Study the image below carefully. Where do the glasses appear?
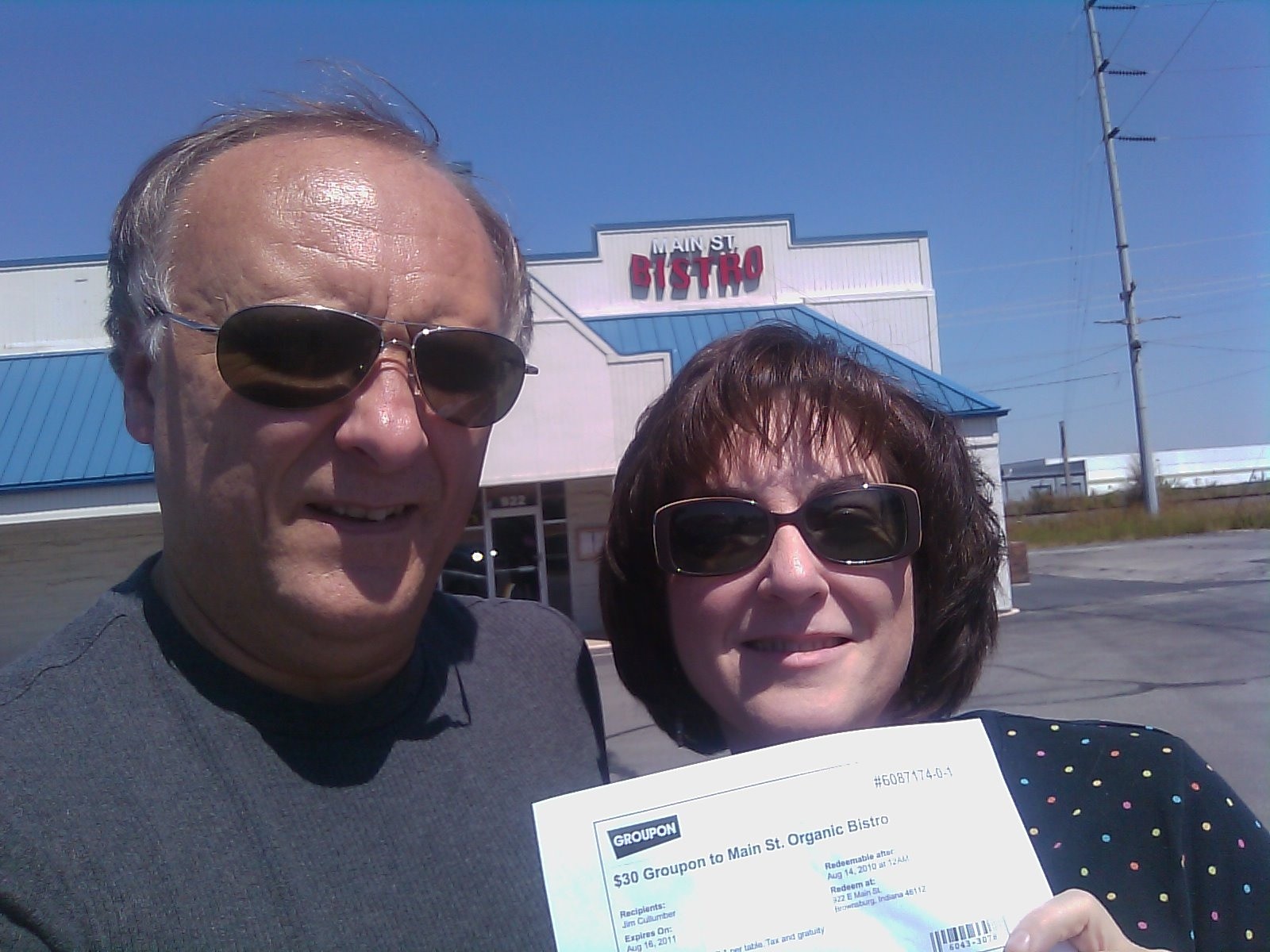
[144,292,537,428]
[646,484,923,578]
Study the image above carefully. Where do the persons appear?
[601,317,1270,952]
[0,68,612,952]
